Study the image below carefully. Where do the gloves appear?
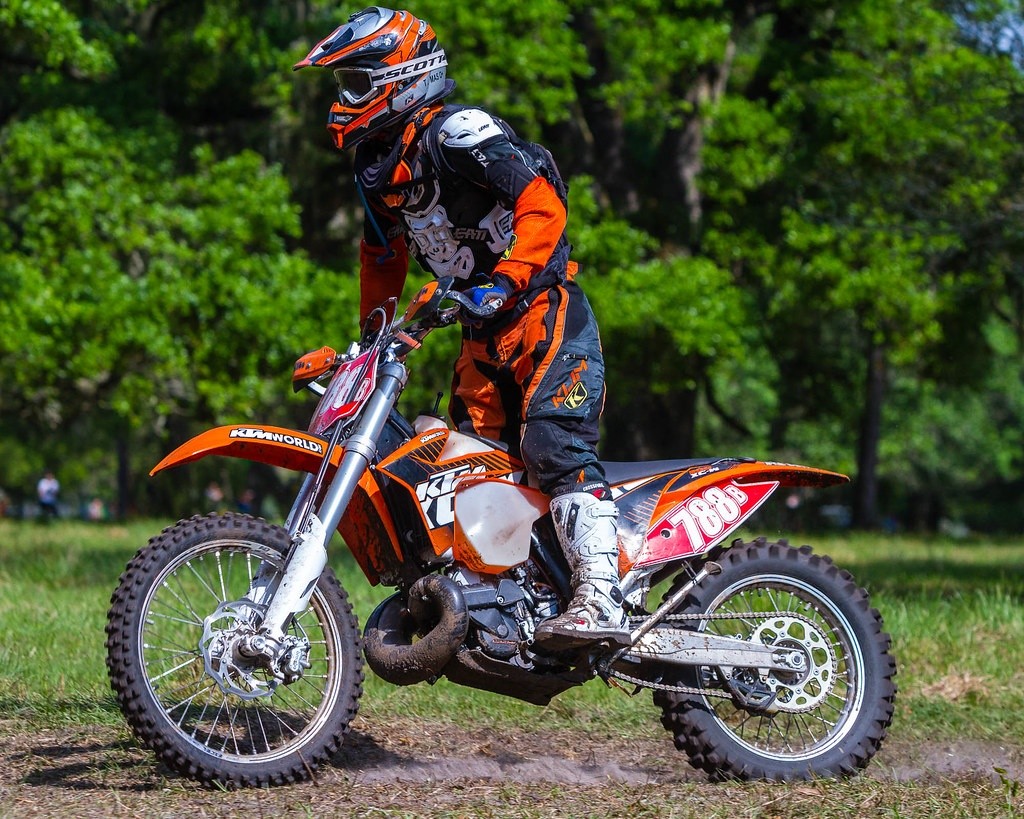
[456,275,516,326]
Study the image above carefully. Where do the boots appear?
[533,492,631,657]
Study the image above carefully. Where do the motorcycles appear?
[105,279,895,791]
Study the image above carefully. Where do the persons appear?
[289,4,633,657]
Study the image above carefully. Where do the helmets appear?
[294,6,447,154]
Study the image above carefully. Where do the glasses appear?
[333,68,381,107]
